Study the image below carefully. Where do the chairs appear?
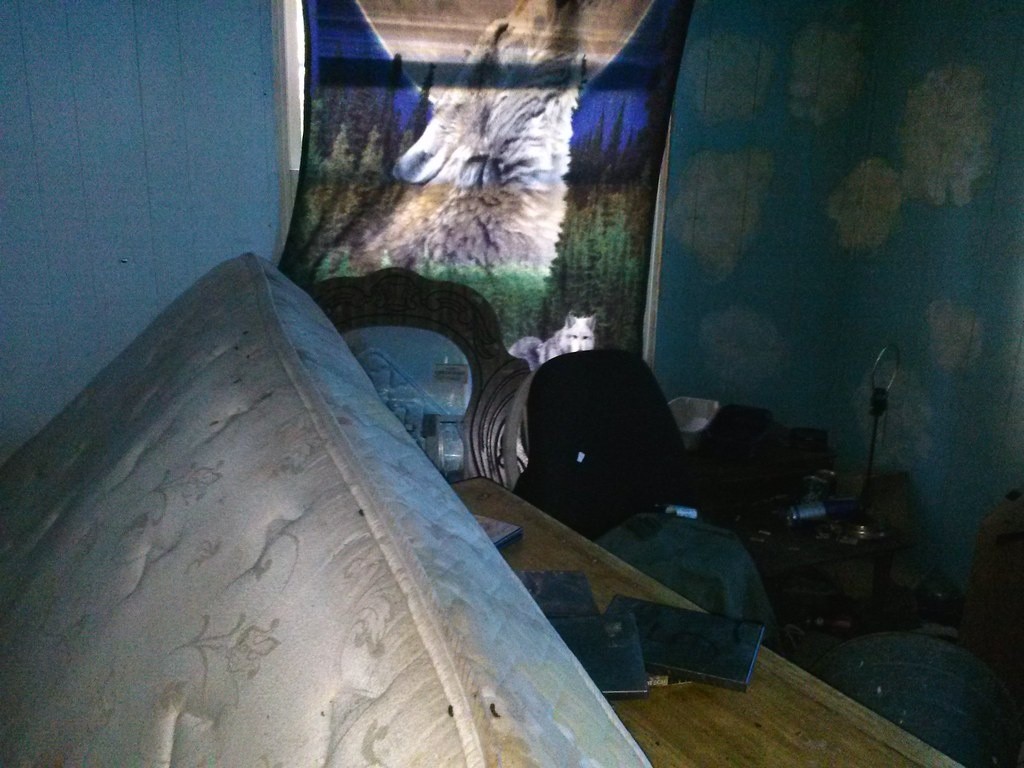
[527,348,691,546]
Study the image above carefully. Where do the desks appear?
[691,451,915,573]
[447,476,968,767]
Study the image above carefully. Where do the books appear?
[473,513,524,547]
[515,568,764,699]
